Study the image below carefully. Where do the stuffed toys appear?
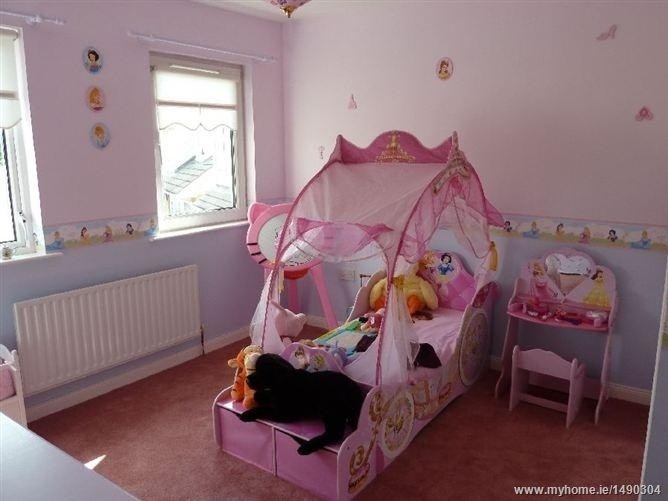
[371,255,440,316]
[227,344,263,409]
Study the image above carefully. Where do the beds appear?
[313,254,502,475]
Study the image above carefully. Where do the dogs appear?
[239,352,369,456]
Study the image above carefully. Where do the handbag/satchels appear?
[413,342,441,368]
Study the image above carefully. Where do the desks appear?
[494,247,618,424]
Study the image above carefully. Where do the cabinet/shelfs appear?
[212,343,380,501]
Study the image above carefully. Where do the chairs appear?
[509,343,587,427]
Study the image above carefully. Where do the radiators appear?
[13,263,204,400]
